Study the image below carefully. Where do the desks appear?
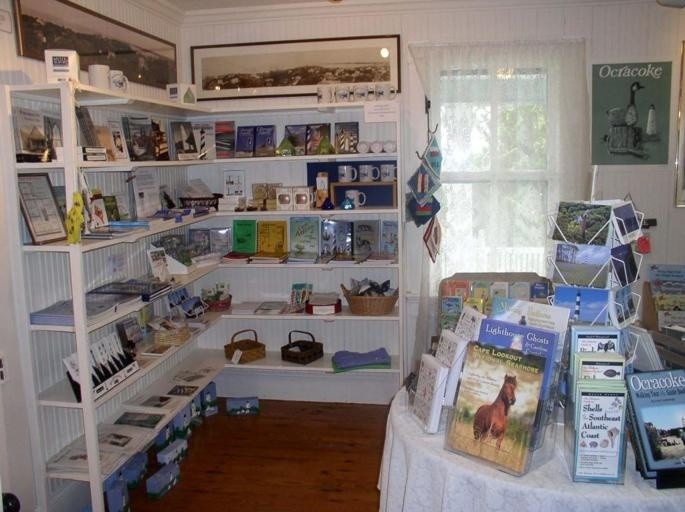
[375,382,685,512]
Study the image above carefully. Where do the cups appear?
[88,64,129,93]
[317,83,397,104]
[338,164,397,209]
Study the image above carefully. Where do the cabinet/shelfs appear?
[2,79,406,512]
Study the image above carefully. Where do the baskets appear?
[280,330,323,365]
[341,283,399,315]
[224,329,265,364]
[154,326,190,346]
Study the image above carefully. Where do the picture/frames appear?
[16,172,67,246]
[12,0,177,89]
[190,33,402,101]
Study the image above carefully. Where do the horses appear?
[471,373,518,456]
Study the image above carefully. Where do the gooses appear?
[604,82,646,127]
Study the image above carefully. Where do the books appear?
[625,326,665,373]
[222,251,397,265]
[11,108,64,163]
[231,301,288,315]
[46,367,214,476]
[215,120,359,158]
[74,108,216,164]
[82,207,210,240]
[411,278,626,486]
[626,368,684,484]
[30,281,173,327]
